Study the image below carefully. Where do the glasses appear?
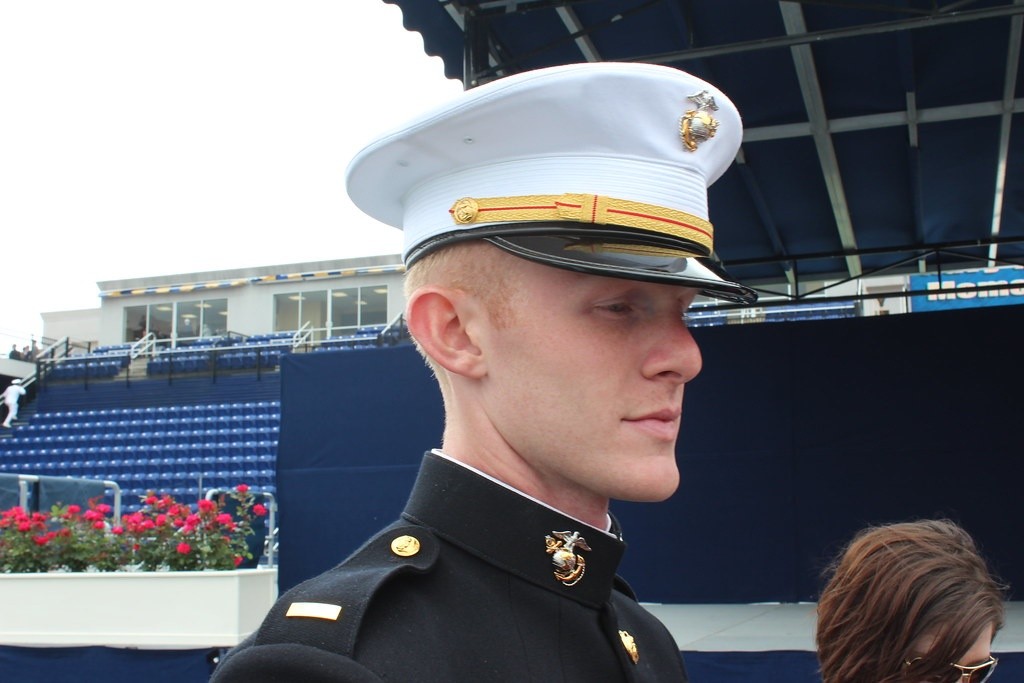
[903,656,999,683]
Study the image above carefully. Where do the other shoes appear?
[13,416,18,419]
[3,423,12,428]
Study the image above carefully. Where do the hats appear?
[11,379,22,384]
[347,59,759,305]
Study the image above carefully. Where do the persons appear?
[815,519,1004,683]
[10,340,38,363]
[207,62,757,683]
[2,379,26,429]
[139,315,211,345]
